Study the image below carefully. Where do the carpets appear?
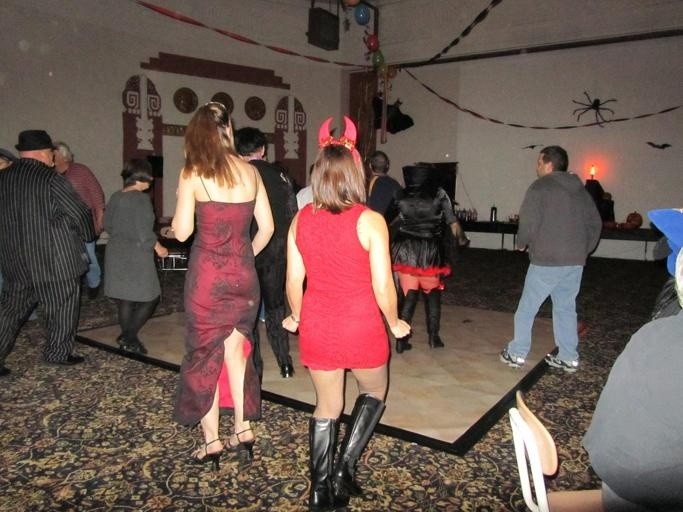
[74,300,590,458]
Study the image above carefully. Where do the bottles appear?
[454,206,477,222]
[490,206,496,222]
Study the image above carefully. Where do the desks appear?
[459,218,663,262]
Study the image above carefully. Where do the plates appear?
[245,96,265,120]
[172,87,197,114]
[210,93,234,115]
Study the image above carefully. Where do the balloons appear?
[347,0,389,75]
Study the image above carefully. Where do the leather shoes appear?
[60,353,82,364]
[279,364,294,378]
[429,337,444,347]
[396,341,412,353]
[116,335,148,354]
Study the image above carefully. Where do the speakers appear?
[306,8,341,51]
[146,155,163,177]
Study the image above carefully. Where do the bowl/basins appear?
[508,215,519,222]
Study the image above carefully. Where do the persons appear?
[579,204,683,512]
[170,101,274,473]
[279,119,412,512]
[388,162,471,354]
[498,144,604,377]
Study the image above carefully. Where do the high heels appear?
[191,439,223,473]
[223,428,256,460]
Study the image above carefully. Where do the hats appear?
[646,203,683,279]
[14,129,57,151]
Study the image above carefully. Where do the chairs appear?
[504,384,562,511]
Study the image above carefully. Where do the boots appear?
[309,417,338,511]
[330,393,387,506]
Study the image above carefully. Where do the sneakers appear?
[499,350,525,368]
[545,353,578,374]
